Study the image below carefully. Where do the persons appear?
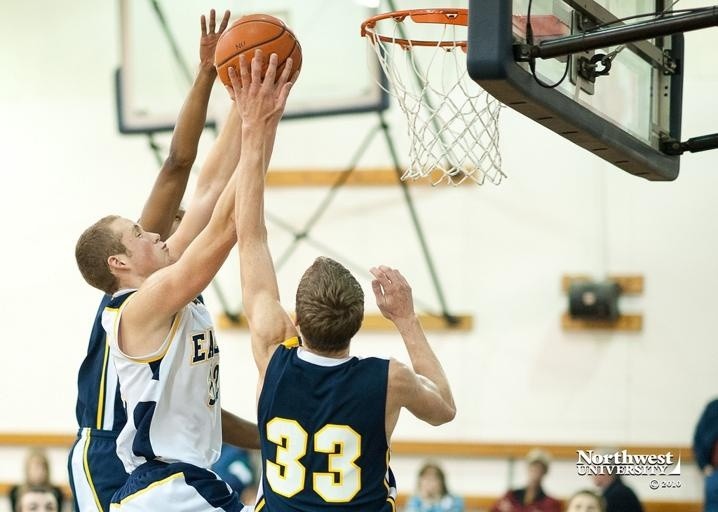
[68,5,263,512]
[72,45,305,512]
[583,448,646,512]
[484,445,565,512]
[562,487,605,512]
[401,458,466,512]
[11,486,62,512]
[6,444,68,512]
[690,398,717,512]
[217,47,461,511]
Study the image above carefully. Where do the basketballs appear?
[215,14,302,88]
